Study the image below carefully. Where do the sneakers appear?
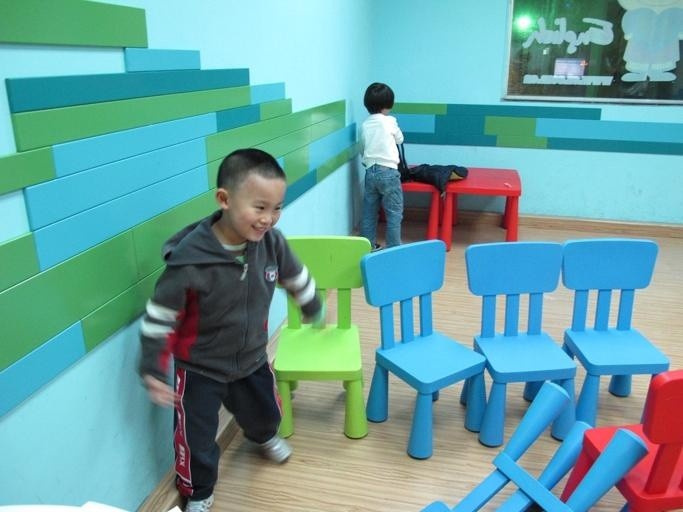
[257,436,292,464]
[371,244,381,252]
[183,493,215,512]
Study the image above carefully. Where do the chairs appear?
[560,241,672,432]
[415,377,648,512]
[360,241,488,463]
[465,240,579,450]
[272,238,372,440]
[555,367,681,512]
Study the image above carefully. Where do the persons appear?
[132,146,326,511]
[355,81,405,253]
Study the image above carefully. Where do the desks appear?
[442,168,520,253]
[377,164,441,241]
